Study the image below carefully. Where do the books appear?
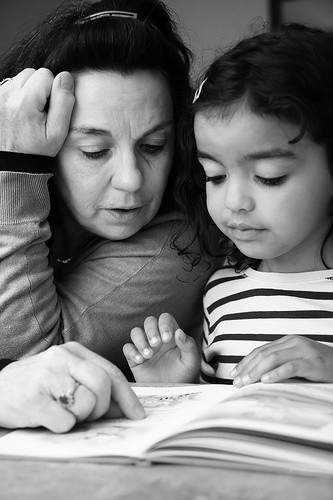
[0,384,333,480]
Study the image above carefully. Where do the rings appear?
[57,380,81,408]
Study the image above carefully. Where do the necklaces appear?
[47,239,78,263]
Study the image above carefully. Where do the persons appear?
[123,22,333,388]
[0,1,233,435]
